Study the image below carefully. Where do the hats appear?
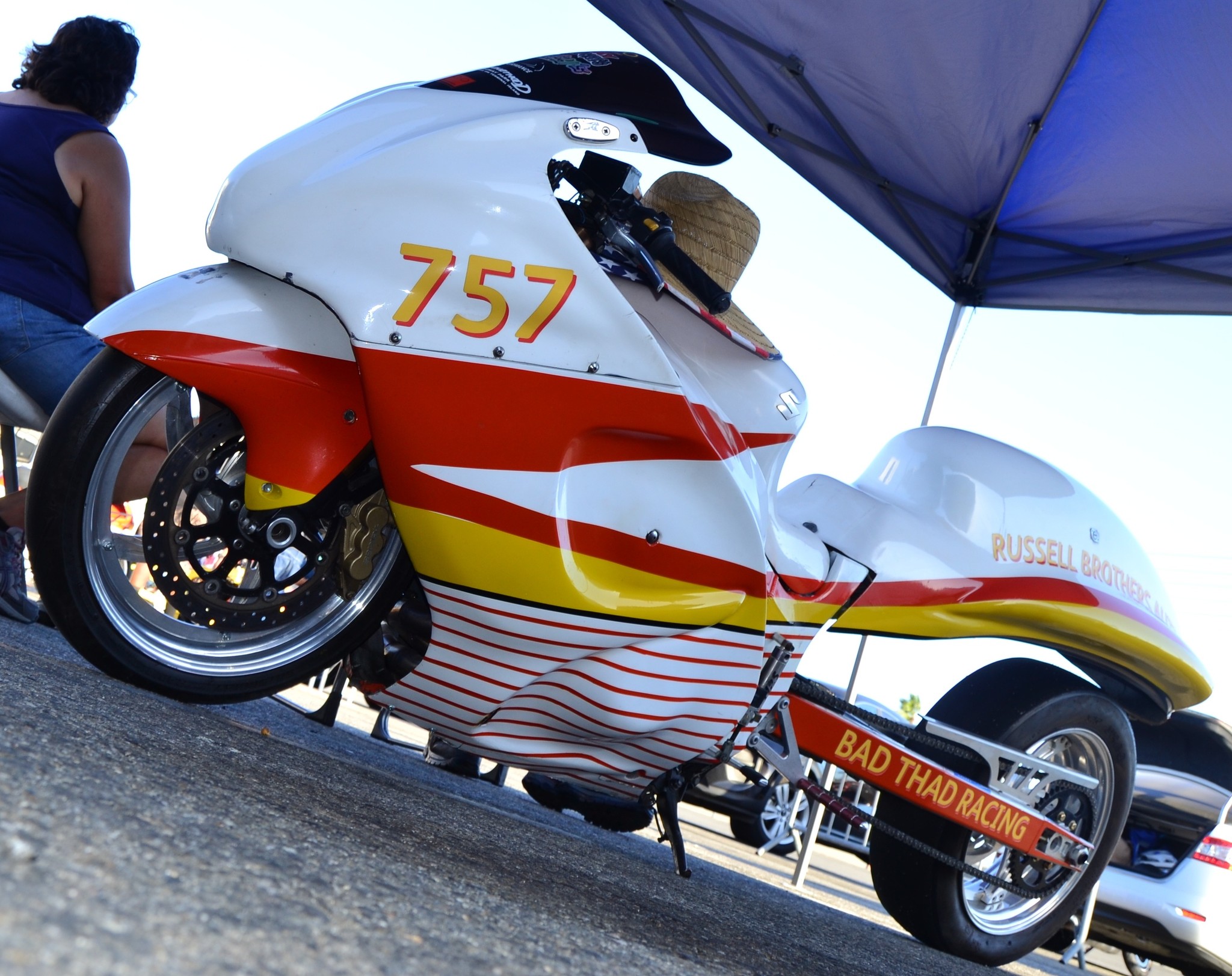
[595,171,784,361]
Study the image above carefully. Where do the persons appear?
[0,17,198,624]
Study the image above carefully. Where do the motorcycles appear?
[19,49,1215,969]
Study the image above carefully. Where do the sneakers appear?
[423,730,481,778]
[0,527,40,624]
[521,771,654,834]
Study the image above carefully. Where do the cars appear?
[1055,708,1232,976]
[695,680,916,856]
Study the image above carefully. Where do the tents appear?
[591,0,1232,890]
